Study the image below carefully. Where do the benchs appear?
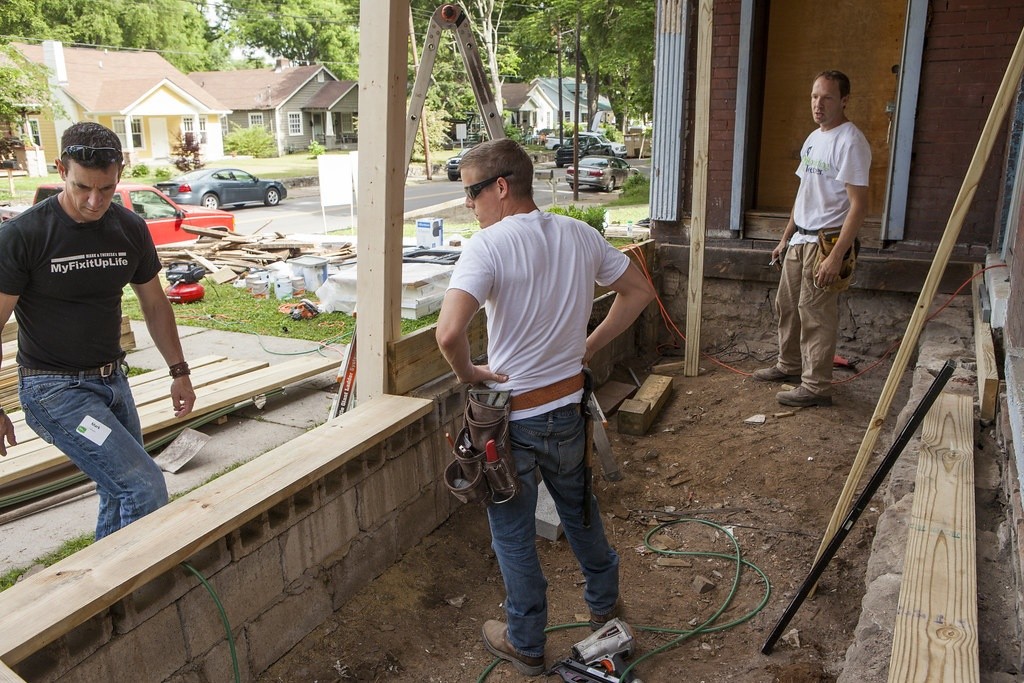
[339,132,358,143]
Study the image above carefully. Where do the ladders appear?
[327,303,357,423]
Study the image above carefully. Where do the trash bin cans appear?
[291,255,330,292]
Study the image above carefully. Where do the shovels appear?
[0,427,212,526]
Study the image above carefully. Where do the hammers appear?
[770,252,783,270]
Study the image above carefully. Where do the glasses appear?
[60,145,124,165]
[463,170,513,200]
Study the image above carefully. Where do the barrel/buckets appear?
[243,268,305,301]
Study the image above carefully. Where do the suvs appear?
[447,142,480,181]
[555,136,613,167]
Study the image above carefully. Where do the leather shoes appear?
[752,365,802,383]
[481,618,546,676]
[589,609,615,632]
[775,385,833,408]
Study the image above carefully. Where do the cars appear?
[155,167,287,209]
[566,154,640,192]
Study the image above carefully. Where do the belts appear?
[17,351,127,378]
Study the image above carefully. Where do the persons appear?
[435,139,652,677]
[0,122,196,542]
[753,70,872,407]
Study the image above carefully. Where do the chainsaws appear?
[289,299,320,321]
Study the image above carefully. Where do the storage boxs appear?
[416,217,443,248]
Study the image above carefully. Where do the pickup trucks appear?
[545,130,627,158]
[32,184,235,245]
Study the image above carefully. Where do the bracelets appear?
[169,362,190,378]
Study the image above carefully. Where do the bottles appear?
[627,220,633,238]
